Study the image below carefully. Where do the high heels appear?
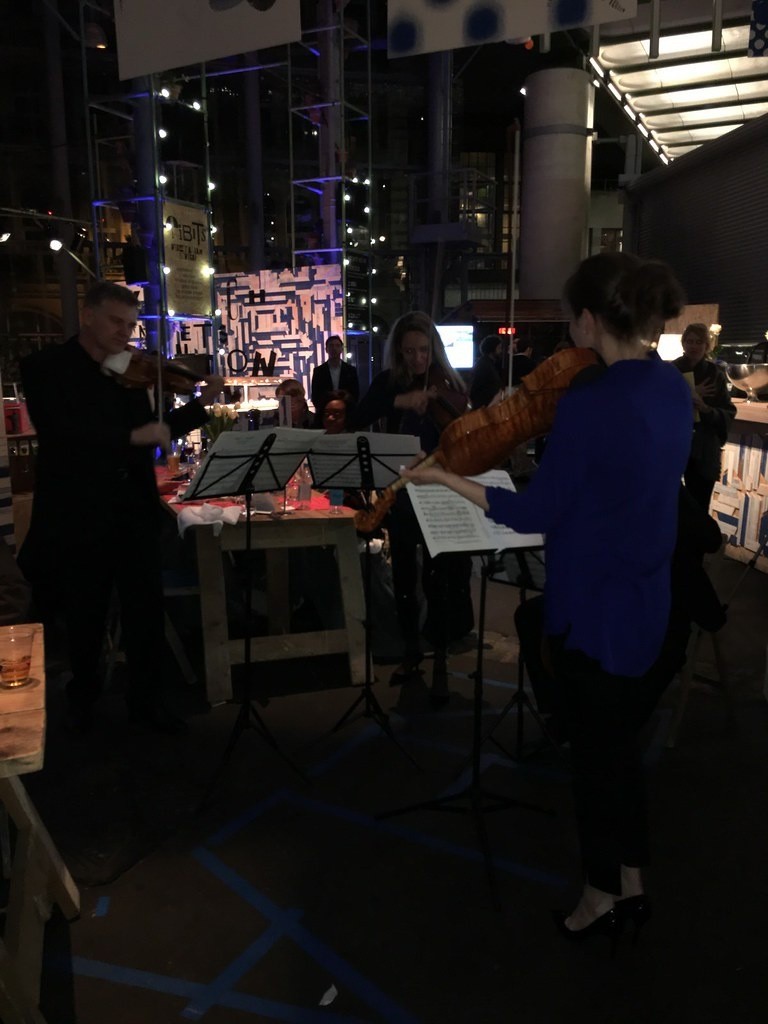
[616,894,654,942]
[549,902,621,960]
[390,651,424,687]
[429,669,450,712]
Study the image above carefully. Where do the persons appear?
[665,321,736,513]
[310,335,360,430]
[354,311,519,715]
[397,252,692,957]
[514,485,730,746]
[495,330,521,396]
[306,391,365,511]
[140,380,160,425]
[510,336,542,390]
[16,279,225,722]
[527,323,583,467]
[468,333,503,413]
[261,377,320,433]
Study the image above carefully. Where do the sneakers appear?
[128,704,192,736]
[64,701,97,735]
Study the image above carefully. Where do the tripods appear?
[190,494,319,818]
[322,489,430,776]
[467,549,557,783]
[370,552,564,920]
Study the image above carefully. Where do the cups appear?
[0,626,37,690]
[186,457,202,480]
[167,443,180,471]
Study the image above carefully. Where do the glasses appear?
[401,345,429,357]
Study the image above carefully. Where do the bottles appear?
[328,489,344,515]
[297,467,313,509]
[240,408,271,509]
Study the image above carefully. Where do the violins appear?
[413,366,468,424]
[107,344,208,394]
[354,344,599,534]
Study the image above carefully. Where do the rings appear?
[416,400,423,406]
[702,382,705,386]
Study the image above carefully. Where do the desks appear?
[164,488,376,703]
